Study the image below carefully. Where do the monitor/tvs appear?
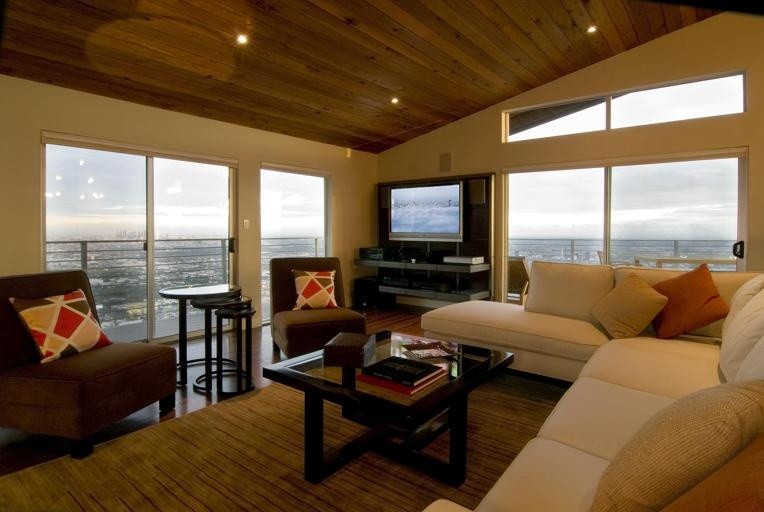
[388,180,464,242]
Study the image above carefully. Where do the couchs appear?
[420,260,764,382]
[267,257,366,362]
[421,341,764,511]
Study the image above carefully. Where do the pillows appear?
[290,268,339,311]
[7,289,112,364]
[591,382,763,512]
[661,427,764,510]
[650,264,730,338]
[592,271,669,339]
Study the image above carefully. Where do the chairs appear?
[1,270,177,460]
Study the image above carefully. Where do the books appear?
[356,356,448,396]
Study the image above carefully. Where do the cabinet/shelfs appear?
[355,248,491,309]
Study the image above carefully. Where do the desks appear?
[158,282,256,396]
